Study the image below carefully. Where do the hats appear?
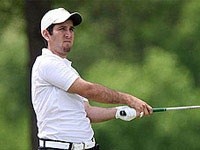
[40,8,82,33]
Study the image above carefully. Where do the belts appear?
[39,138,96,150]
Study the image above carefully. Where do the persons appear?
[30,7,154,150]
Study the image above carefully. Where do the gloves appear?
[114,105,144,122]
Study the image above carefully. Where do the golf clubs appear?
[119,105,200,116]
